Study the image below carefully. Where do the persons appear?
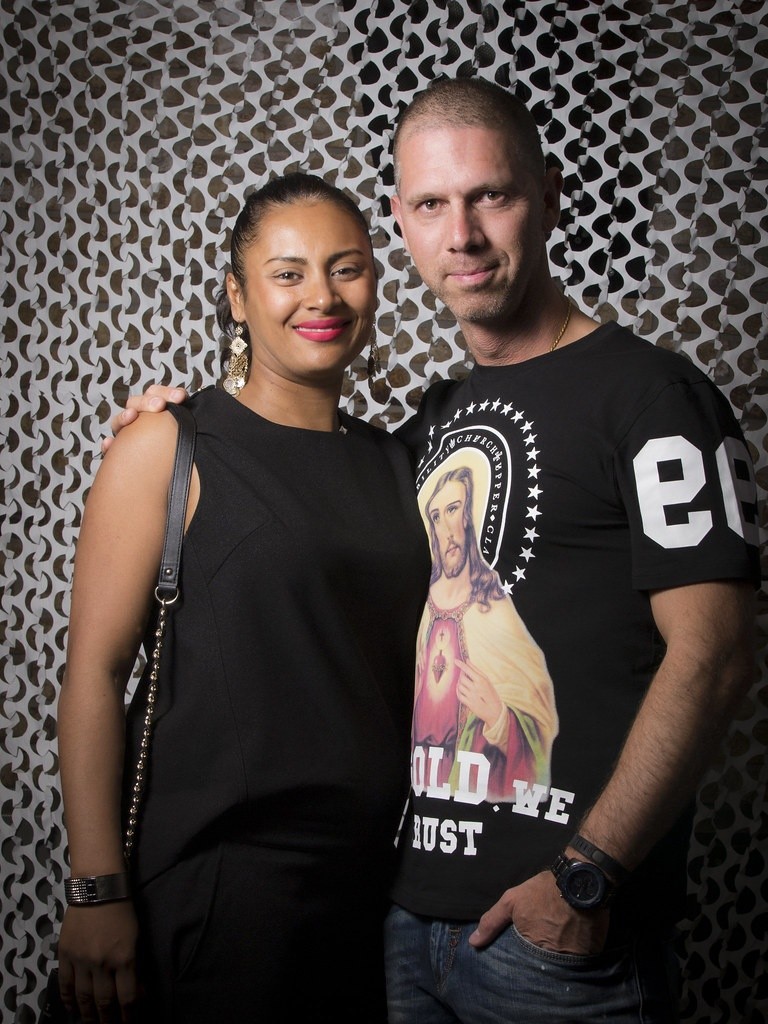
[48,172,432,1024]
[383,77,767,1024]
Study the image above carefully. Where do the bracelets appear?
[65,874,129,906]
[569,834,632,883]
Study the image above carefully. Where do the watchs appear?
[551,846,624,914]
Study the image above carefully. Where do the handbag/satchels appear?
[33,969,120,1023]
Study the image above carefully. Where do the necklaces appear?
[550,295,572,354]
